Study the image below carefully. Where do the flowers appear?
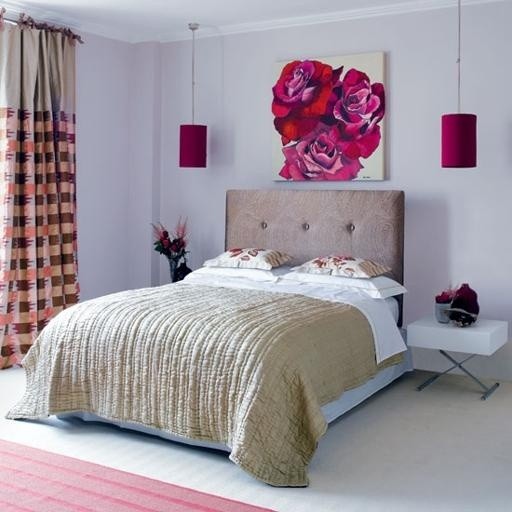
[434,279,462,304]
[148,213,191,284]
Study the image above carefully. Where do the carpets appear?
[0,435,281,512]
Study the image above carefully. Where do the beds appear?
[3,188,411,491]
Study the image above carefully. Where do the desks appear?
[405,313,510,402]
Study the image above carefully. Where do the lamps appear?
[438,1,478,169]
[177,22,210,169]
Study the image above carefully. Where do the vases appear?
[434,303,451,324]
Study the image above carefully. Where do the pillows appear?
[184,247,409,301]
